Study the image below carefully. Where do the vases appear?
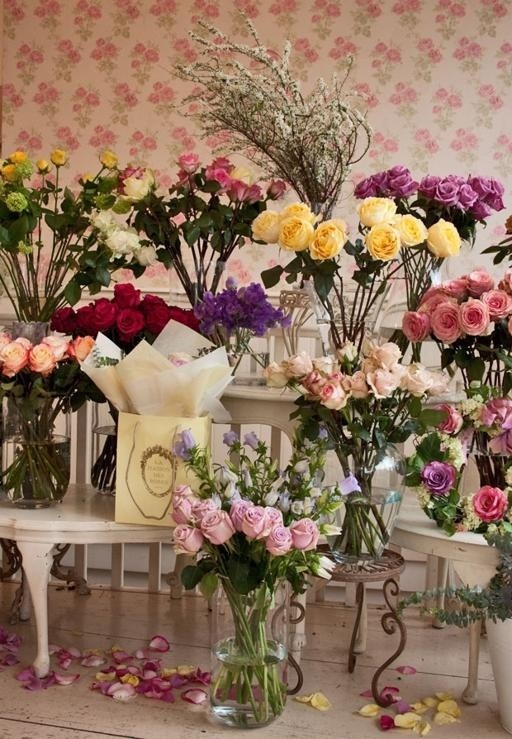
[483,617,512,734]
[206,637,290,728]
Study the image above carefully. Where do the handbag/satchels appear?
[111,412,214,533]
